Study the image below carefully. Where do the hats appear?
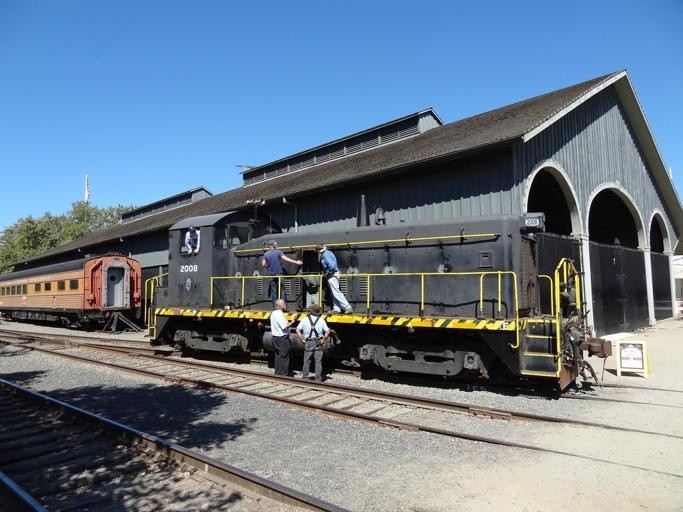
[315,244,324,251]
[266,240,276,246]
[188,226,195,231]
[309,304,321,316]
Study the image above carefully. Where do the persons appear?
[314,242,353,315]
[269,298,300,375]
[296,303,331,383]
[183,227,200,256]
[262,240,304,308]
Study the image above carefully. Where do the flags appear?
[84,174,90,204]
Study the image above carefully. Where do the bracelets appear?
[291,319,294,323]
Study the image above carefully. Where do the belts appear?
[307,337,324,341]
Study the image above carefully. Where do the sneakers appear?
[327,309,341,314]
[345,307,353,315]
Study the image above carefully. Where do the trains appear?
[140,208,612,395]
[0,254,143,334]
